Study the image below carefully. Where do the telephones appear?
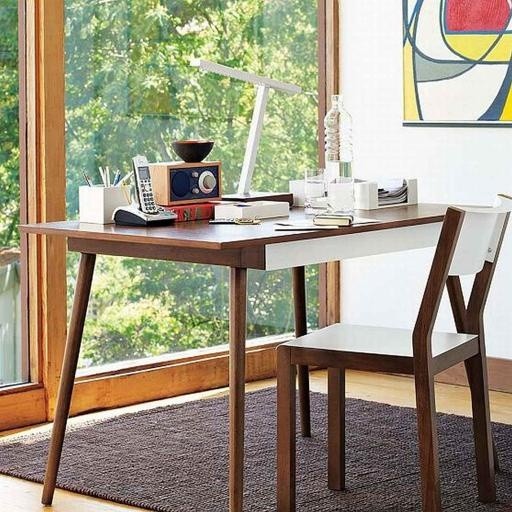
[111,154,178,226]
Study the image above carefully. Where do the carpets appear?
[1,386,510,512]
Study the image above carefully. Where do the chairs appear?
[277,194,512,511]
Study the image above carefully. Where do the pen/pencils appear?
[84,165,135,188]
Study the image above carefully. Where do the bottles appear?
[322,93,354,185]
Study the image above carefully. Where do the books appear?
[378,183,407,205]
[312,212,354,226]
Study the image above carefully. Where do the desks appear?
[15,201,499,511]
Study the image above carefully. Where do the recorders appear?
[147,160,224,206]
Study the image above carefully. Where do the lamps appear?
[189,58,302,208]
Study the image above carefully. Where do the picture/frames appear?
[402,0,512,126]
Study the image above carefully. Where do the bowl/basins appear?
[172,140,215,163]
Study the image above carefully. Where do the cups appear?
[327,178,356,217]
[305,168,328,214]
